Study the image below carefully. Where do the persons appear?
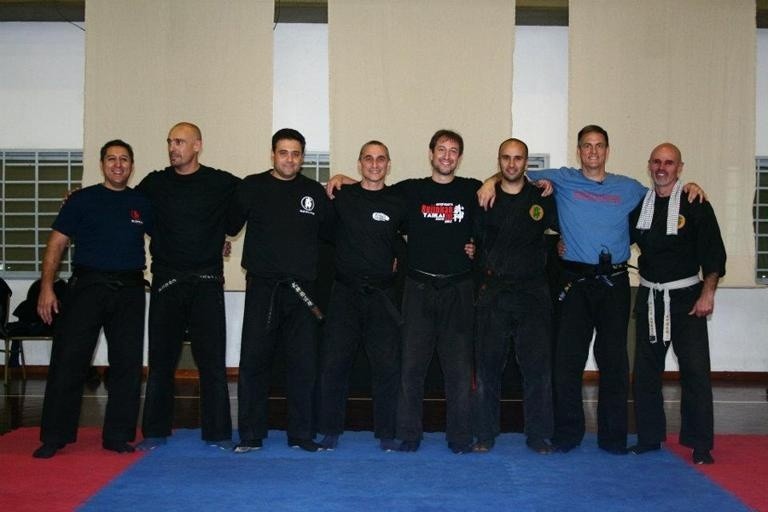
[317,140,477,452]
[59,120,244,452]
[30,138,232,460]
[469,137,561,456]
[224,126,398,454]
[325,128,554,454]
[477,124,707,458]
[556,142,728,466]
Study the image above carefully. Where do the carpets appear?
[2,424,768,512]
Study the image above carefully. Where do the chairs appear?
[0,280,65,405]
